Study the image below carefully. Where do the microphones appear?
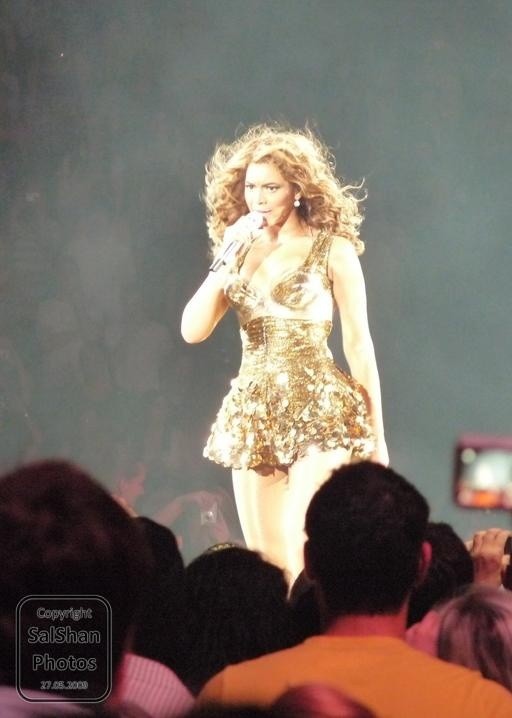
[208,211,264,272]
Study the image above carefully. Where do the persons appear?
[177,123,390,603]
[0,449,510,715]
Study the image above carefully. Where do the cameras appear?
[200,501,218,526]
